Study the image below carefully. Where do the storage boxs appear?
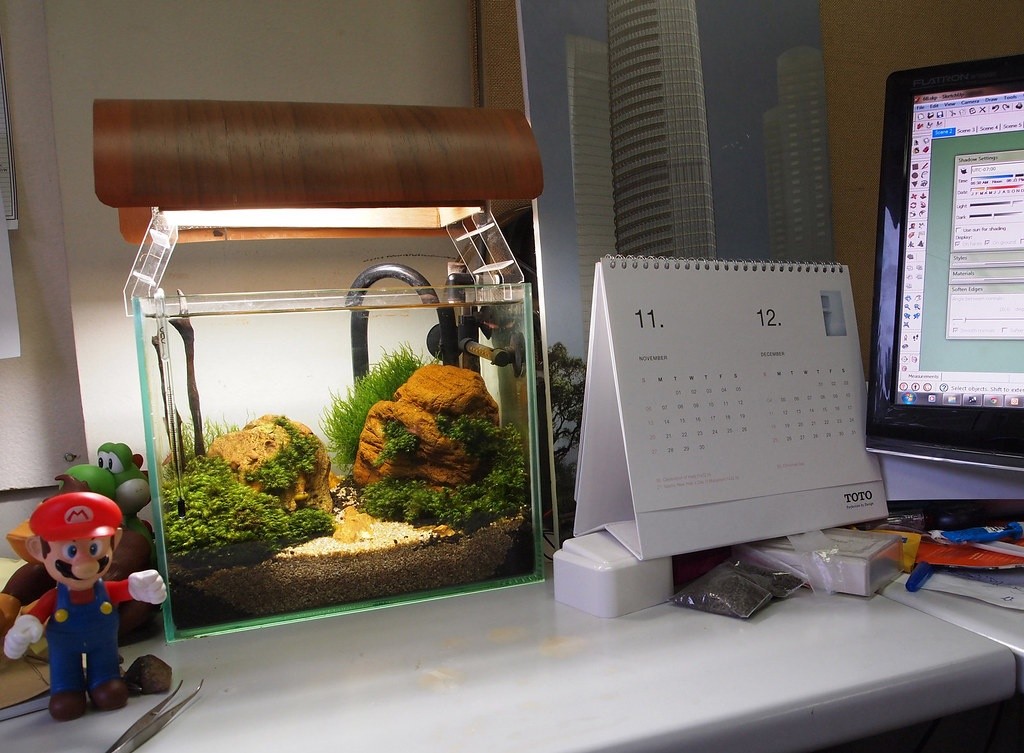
[88,95,544,645]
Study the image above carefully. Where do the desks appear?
[876,582,1024,753]
[0,590,1018,753]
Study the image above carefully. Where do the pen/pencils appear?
[905,559,933,592]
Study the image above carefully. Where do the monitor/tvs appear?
[866,54,1024,474]
